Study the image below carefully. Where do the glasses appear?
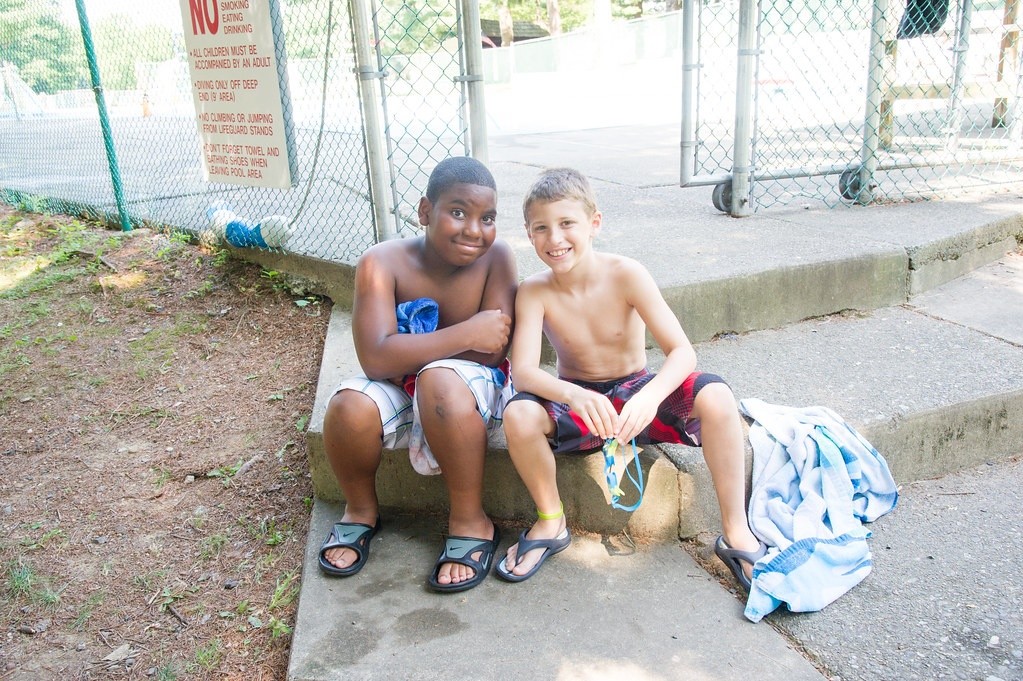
[602,436,643,512]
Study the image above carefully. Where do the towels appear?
[396,297,439,334]
[739,397,900,623]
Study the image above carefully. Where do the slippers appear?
[494,527,572,582]
[714,533,770,591]
[318,510,381,576]
[429,523,501,593]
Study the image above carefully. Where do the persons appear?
[496,167,772,591]
[317,157,518,592]
